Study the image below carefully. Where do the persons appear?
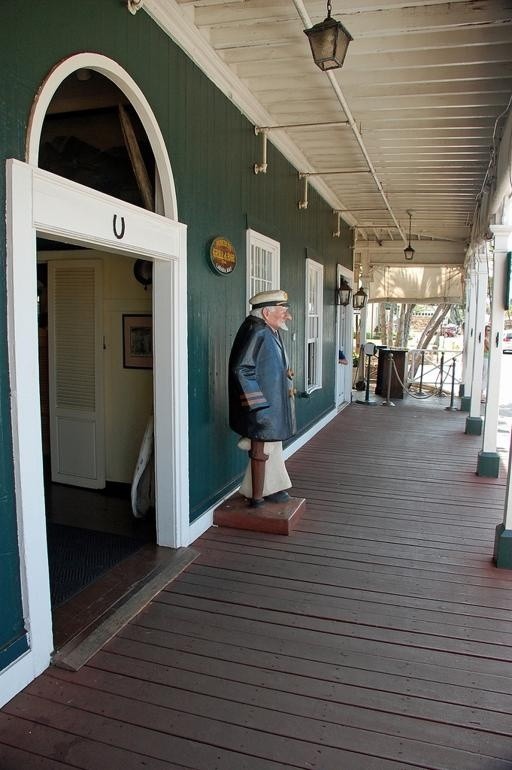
[229,289,296,503]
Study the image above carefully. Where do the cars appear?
[503,330,512,353]
[428,326,456,337]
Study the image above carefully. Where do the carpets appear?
[47,522,149,613]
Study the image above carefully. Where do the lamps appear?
[334,281,352,306]
[404,213,415,260]
[303,0,351,73]
[352,286,367,309]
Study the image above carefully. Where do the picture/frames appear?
[122,313,154,370]
